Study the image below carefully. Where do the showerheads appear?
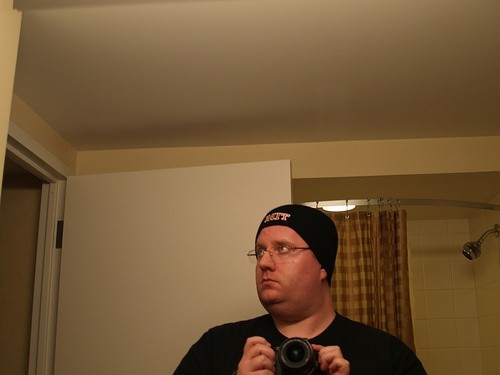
[460,224,500,262]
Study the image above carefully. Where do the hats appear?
[255,205,338,286]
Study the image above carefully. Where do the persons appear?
[173,204,428,375]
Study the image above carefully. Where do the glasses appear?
[246,243,310,264]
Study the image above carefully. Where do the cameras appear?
[271,337,326,375]
